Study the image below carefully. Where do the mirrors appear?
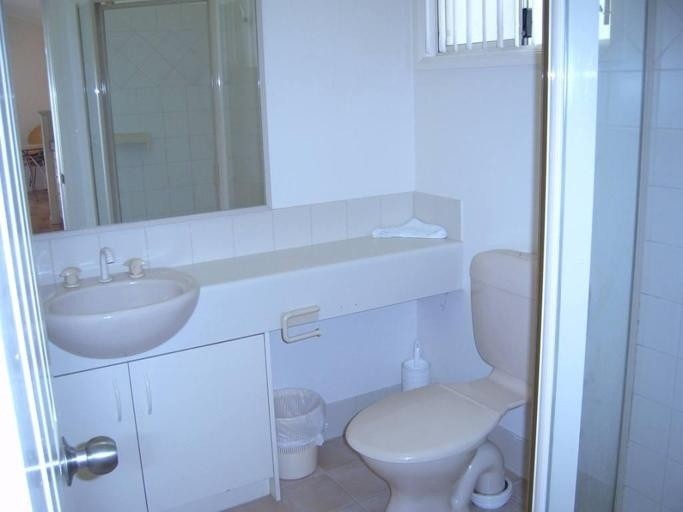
[0,4,272,231]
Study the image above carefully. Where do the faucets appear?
[98,247,115,283]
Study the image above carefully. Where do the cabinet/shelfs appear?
[50,332,270,510]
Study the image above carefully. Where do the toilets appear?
[344,248,543,512]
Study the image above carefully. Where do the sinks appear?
[44,267,198,358]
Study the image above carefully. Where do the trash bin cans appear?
[273,387,323,480]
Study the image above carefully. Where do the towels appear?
[370,218,447,240]
